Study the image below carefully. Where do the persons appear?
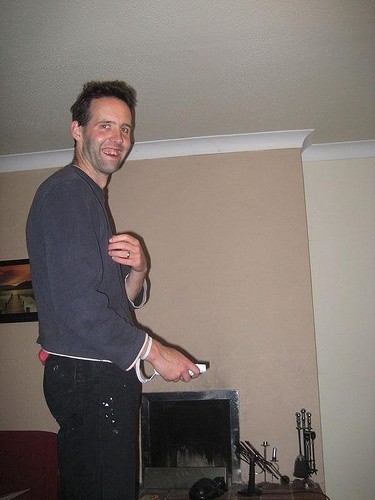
[23,77,202,500]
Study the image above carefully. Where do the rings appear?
[126,252,130,258]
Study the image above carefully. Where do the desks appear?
[139,482,327,500]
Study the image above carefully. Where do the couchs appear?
[0,429,58,500]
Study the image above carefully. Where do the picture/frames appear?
[0,258,40,323]
